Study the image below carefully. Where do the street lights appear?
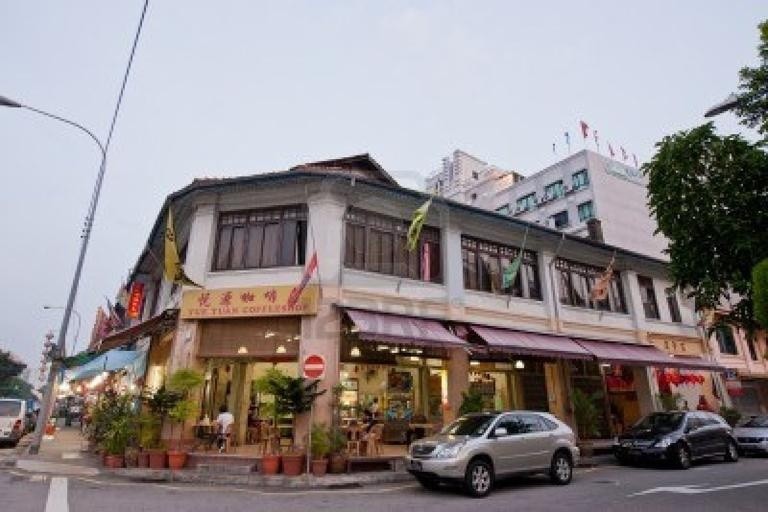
[0,97,108,458]
[42,305,82,360]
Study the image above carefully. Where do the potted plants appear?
[91,368,203,468]
[253,368,344,475]
[569,390,604,458]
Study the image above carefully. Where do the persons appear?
[216,405,234,452]
[367,397,378,432]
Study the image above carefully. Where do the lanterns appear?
[38,333,54,382]
[656,372,705,388]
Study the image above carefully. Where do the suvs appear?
[402,409,580,499]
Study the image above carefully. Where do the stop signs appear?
[303,356,326,380]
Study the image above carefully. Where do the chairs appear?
[218,424,236,452]
[407,415,426,446]
[347,421,384,457]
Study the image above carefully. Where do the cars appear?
[0,396,37,446]
[732,413,767,457]
[612,408,738,468]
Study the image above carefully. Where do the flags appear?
[552,121,638,167]
[406,197,433,253]
[165,208,204,288]
[288,255,317,308]
[502,226,528,289]
[588,255,616,301]
